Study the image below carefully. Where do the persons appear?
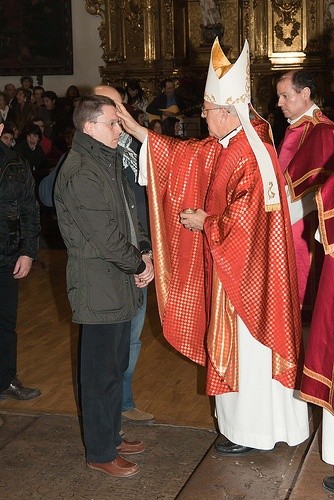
[116,36,309,457]
[40,85,155,420]
[0,76,181,173]
[0,112,42,401]
[54,95,144,478]
[276,69,334,400]
[284,123,334,495]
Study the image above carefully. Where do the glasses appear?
[200,106,230,115]
[90,121,121,129]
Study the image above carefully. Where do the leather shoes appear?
[0,375,41,400]
[116,440,145,454]
[87,456,140,477]
[215,440,254,456]
[323,476,334,494]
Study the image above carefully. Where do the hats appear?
[204,36,251,105]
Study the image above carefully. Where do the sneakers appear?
[120,408,155,424]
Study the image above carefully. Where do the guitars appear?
[148,102,204,125]
